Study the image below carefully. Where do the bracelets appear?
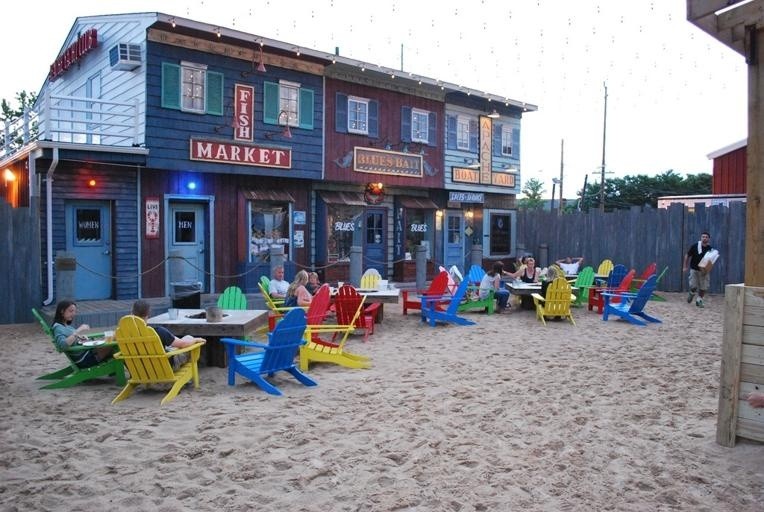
[73,333,80,339]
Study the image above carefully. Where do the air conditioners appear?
[108,42,141,71]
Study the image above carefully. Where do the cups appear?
[104,331,113,344]
[329,287,338,295]
[168,308,178,320]
[338,282,344,288]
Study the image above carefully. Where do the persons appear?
[503,257,540,310]
[542,264,561,321]
[268,265,290,297]
[683,232,712,308]
[51,299,115,369]
[519,256,528,269]
[284,269,312,313]
[131,300,207,385]
[478,261,512,314]
[306,272,321,295]
[555,256,584,275]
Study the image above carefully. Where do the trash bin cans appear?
[168,281,203,309]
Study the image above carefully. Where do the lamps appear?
[502,162,517,171]
[464,158,480,167]
[214,102,239,132]
[265,110,293,140]
[487,105,500,118]
[369,133,425,156]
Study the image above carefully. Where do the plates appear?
[82,341,105,346]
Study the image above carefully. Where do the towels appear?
[697,240,703,254]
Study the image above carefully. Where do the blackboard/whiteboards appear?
[488,211,513,257]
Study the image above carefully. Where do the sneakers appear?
[504,308,512,311]
[687,293,695,303]
[696,298,706,307]
[500,310,512,314]
